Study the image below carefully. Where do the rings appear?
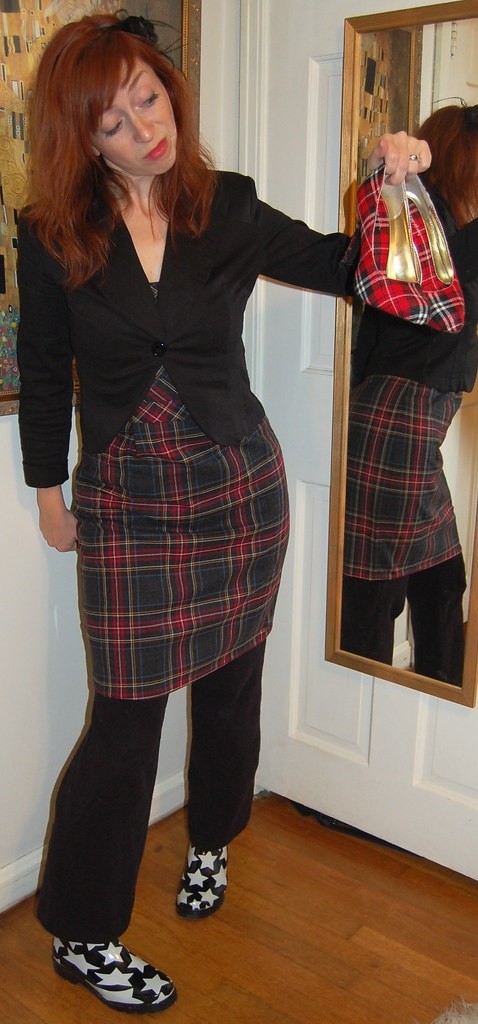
[410,155,419,161]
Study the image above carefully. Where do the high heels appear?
[403,174,465,335]
[339,163,432,331]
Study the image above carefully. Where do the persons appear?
[15,12,431,1015]
[340,95,477,688]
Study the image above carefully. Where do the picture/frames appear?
[0,0,203,419]
[355,20,422,183]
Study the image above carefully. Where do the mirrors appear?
[325,1,478,709]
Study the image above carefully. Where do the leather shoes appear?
[175,844,228,919]
[53,936,177,1013]
